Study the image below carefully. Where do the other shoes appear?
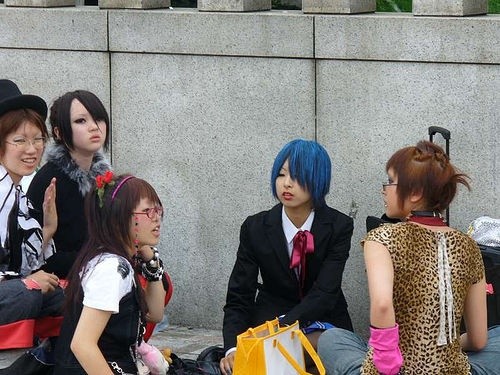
[197,345,225,363]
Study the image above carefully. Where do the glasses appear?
[131,206,163,219]
[381,183,399,191]
[4,135,46,151]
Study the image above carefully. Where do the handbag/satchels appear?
[165,352,222,375]
[232,316,326,375]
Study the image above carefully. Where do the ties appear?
[3,189,22,280]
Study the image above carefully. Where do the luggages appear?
[366,126,451,234]
[460,243,500,335]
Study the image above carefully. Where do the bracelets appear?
[140,247,164,282]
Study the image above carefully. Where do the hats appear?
[0,79,48,125]
[468,216,500,248]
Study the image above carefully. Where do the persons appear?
[0,171,166,375]
[318,141,500,375]
[0,80,68,320]
[220,138,354,375]
[26,90,109,278]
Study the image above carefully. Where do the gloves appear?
[136,341,170,375]
